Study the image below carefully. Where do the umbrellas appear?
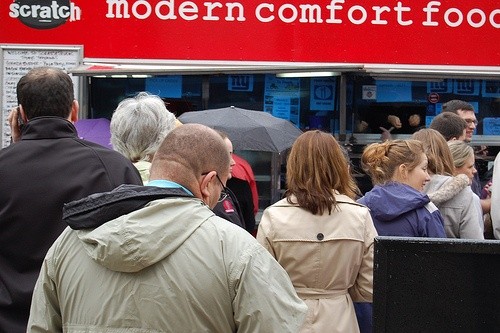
[176,105,304,155]
[73,118,113,148]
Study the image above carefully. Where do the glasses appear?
[201,171,230,202]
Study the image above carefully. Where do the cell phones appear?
[17,107,24,135]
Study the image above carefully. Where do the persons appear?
[23,92,500,333]
[0,66,143,333]
